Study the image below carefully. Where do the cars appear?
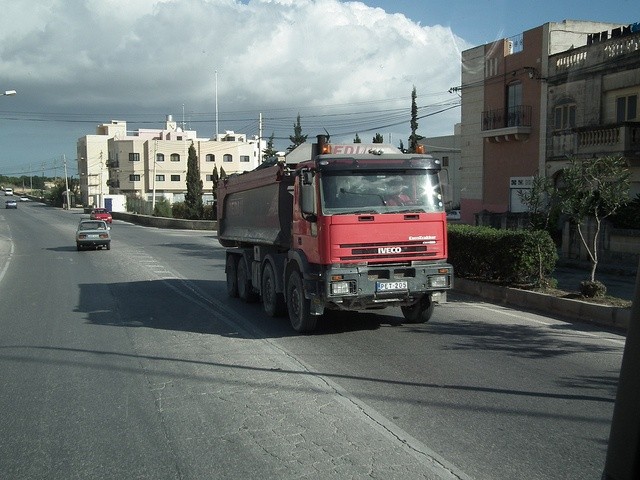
[90,208,112,223]
[20,196,29,202]
[76,220,111,250]
[5,200,17,208]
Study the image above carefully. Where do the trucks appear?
[217,135,452,332]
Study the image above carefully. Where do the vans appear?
[5,188,13,196]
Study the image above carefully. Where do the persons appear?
[97,223,104,230]
[385,176,415,206]
